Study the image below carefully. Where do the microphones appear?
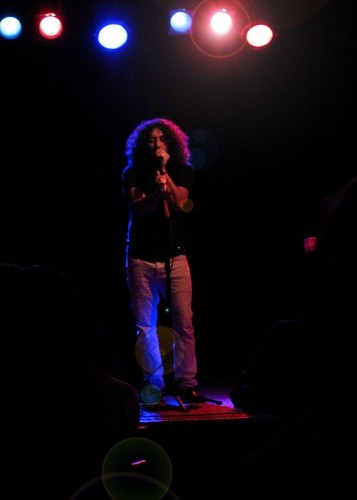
[153,151,166,175]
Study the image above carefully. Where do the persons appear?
[123,118,207,407]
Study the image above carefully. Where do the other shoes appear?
[183,385,208,405]
[143,388,168,410]
